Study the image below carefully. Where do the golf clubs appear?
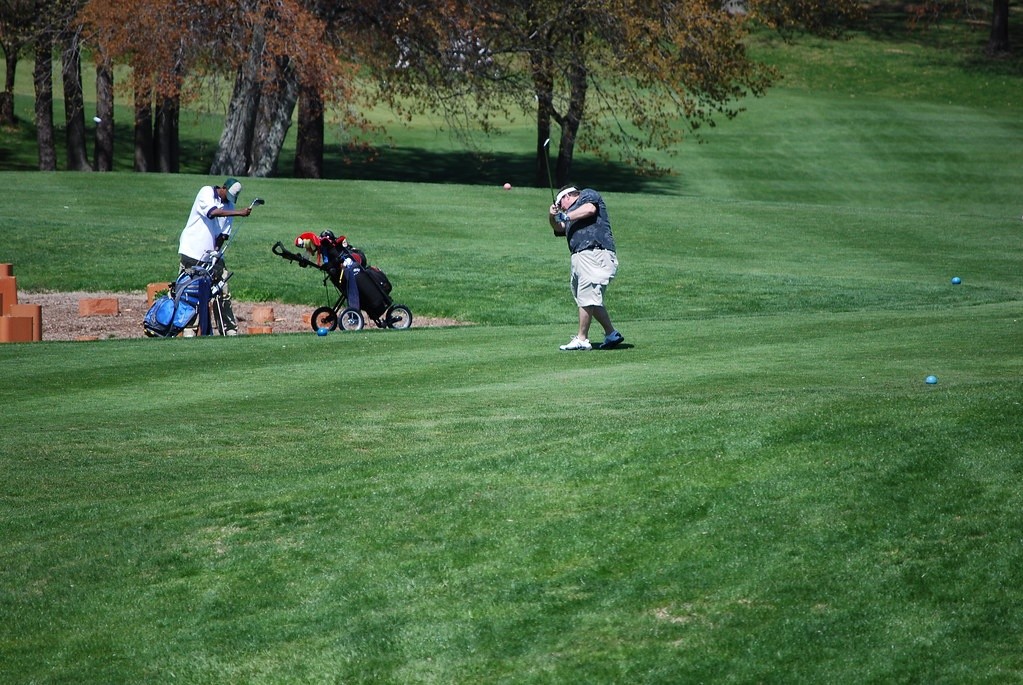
[543,137,557,208]
[207,196,265,273]
[196,250,224,277]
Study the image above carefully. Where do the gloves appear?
[554,213,570,222]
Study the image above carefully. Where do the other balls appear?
[926,376,937,385]
[317,327,328,336]
[503,183,511,190]
[951,277,962,284]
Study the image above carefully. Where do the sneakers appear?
[184,328,196,337]
[225,330,237,337]
[560,336,593,350]
[599,330,624,349]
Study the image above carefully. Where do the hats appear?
[225,178,241,203]
[554,187,578,204]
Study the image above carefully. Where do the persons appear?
[549,185,624,350]
[176,178,251,337]
